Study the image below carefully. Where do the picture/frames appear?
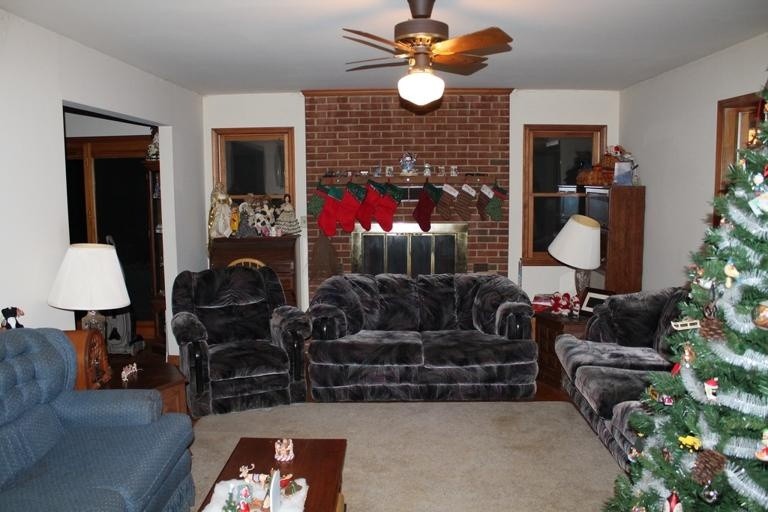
[580,287,617,318]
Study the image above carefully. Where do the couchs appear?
[555,285,691,475]
[305,271,541,402]
[0,327,197,512]
[172,265,311,418]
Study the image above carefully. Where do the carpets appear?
[190,400,628,511]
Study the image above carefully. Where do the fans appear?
[341,1,513,66]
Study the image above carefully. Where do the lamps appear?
[547,214,602,300]
[396,47,445,107]
[47,243,132,329]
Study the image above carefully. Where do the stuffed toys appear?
[549,291,581,318]
[2,306,24,331]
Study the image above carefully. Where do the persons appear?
[208,181,302,240]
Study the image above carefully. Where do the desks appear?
[96,349,188,416]
[535,310,590,389]
[209,233,302,307]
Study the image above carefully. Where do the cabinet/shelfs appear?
[583,184,646,295]
